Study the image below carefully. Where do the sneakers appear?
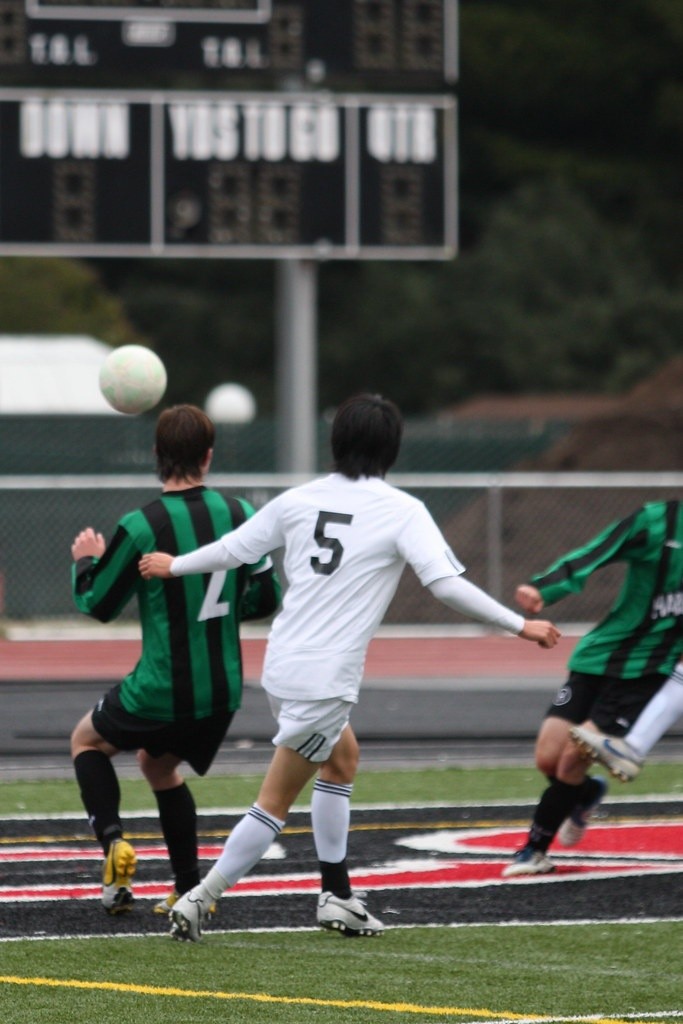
[102,839,138,914]
[558,776,607,847]
[502,846,556,876]
[151,892,216,918]
[568,726,646,782]
[168,890,208,944]
[316,890,386,937]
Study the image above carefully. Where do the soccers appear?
[97,343,169,416]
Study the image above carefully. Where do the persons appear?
[139,394,561,943]
[568,655,683,782]
[501,498,683,877]
[70,405,284,919]
